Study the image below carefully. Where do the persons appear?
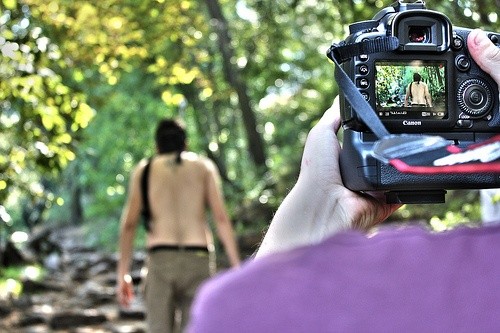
[183,28,500,333]
[405,73,433,108]
[115,118,241,333]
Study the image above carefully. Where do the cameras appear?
[334,0,500,203]
